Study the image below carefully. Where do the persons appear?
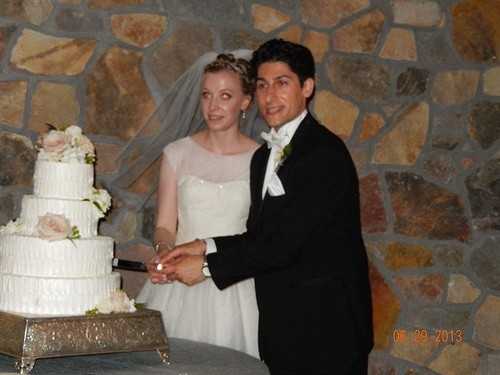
[136,52,264,361]
[159,39,375,375]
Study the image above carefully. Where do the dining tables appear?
[2,337,270,375]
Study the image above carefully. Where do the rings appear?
[161,273,167,282]
[167,279,173,283]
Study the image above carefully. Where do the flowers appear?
[85,287,146,317]
[34,212,81,246]
[82,187,113,218]
[34,121,96,164]
[274,143,292,168]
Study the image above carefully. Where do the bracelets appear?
[155,241,173,252]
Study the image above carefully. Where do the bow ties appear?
[261,130,290,149]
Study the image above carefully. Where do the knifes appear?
[112,257,149,272]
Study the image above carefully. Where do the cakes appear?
[1,125,122,318]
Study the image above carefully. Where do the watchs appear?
[201,252,212,278]
